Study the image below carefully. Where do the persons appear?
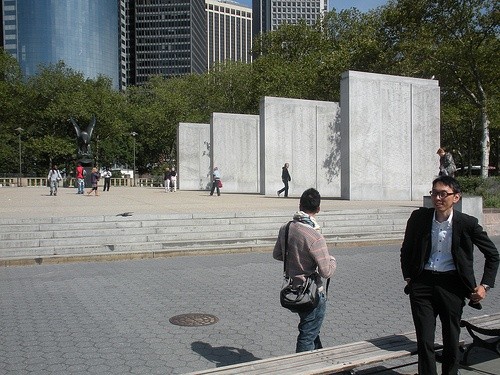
[101,167,112,192]
[272,187,337,353]
[87,167,101,196]
[278,163,292,199]
[169,167,179,192]
[163,167,170,193]
[436,148,457,179]
[398,175,500,375]
[46,164,63,196]
[75,162,85,195]
[208,167,223,197]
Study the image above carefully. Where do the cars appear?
[455,166,497,177]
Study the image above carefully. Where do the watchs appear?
[483,283,491,292]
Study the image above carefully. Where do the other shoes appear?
[277,191,280,196]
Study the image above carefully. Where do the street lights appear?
[16,128,25,187]
[131,131,138,185]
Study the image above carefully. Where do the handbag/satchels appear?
[280,274,319,312]
[218,181,222,187]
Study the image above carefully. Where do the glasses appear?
[429,190,457,199]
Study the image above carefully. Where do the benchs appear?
[137,181,163,187]
[460,307,500,367]
[179,312,466,375]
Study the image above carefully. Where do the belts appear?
[422,269,457,275]
